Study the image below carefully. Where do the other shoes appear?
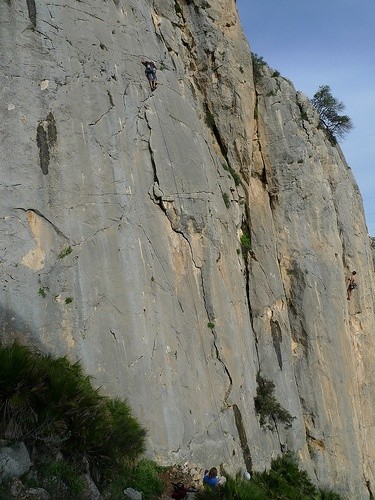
[149,86,156,91]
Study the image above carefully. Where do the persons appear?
[141,58,158,91]
[203,468,223,490]
[346,270,358,300]
